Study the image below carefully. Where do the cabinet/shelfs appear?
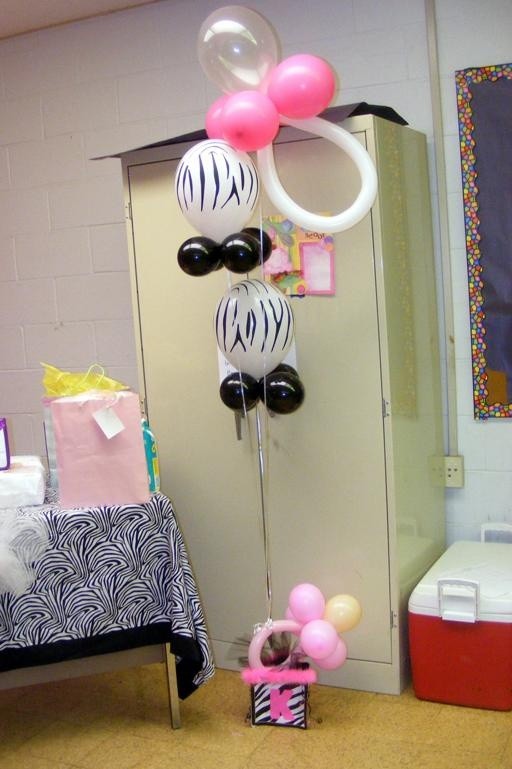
[118,106,449,700]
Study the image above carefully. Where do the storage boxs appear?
[409,540,512,715]
[250,659,311,729]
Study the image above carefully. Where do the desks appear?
[0,486,217,731]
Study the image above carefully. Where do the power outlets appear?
[444,456,464,488]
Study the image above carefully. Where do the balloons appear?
[248,580,362,671]
